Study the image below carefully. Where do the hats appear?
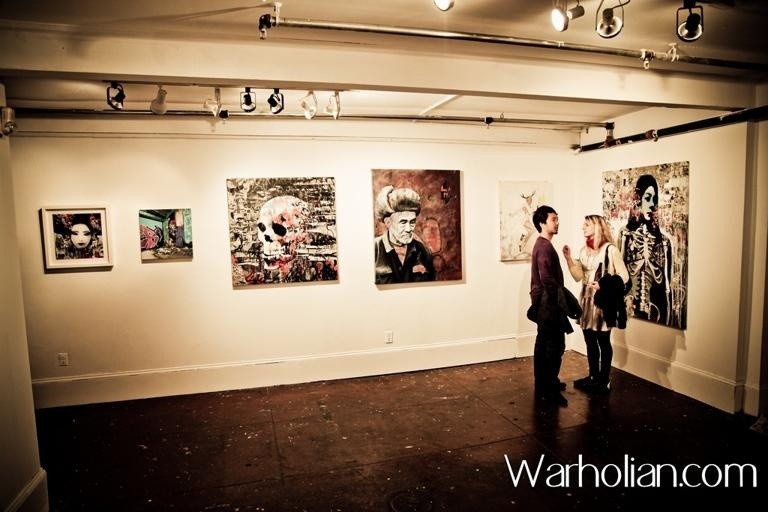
[374,186,422,221]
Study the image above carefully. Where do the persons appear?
[525,204,581,408]
[617,173,678,325]
[560,213,630,397]
[54,215,102,259]
[373,184,438,285]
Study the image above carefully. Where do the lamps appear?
[105,80,342,120]
[549,1,706,43]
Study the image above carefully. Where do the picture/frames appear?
[42,204,113,269]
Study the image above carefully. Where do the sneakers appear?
[552,391,568,407]
[574,377,592,388]
[560,383,567,387]
